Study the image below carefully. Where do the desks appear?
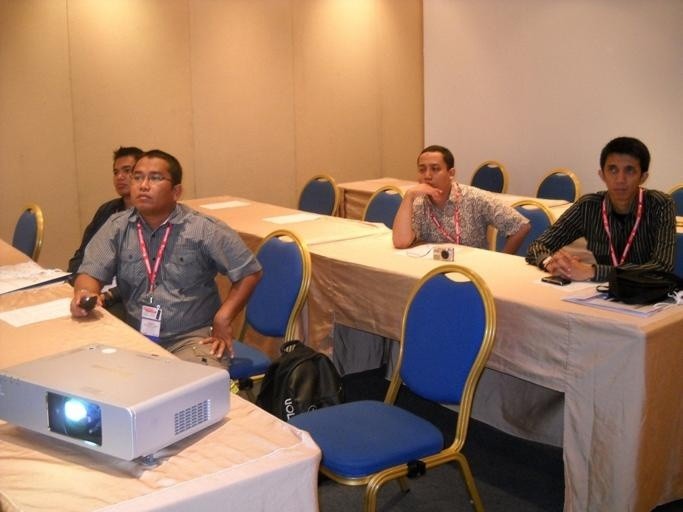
[308,232,681,509]
[338,174,521,221]
[1,273,319,507]
[486,200,682,264]
[2,239,45,291]
[180,195,389,346]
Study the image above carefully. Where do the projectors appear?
[1,344,231,461]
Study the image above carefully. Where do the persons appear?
[70,148,264,369]
[66,145,145,320]
[392,143,532,256]
[524,134,678,282]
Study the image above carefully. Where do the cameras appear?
[432,246,454,262]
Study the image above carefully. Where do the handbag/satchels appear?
[605,263,683,305]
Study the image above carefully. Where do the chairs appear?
[13,205,45,263]
[469,162,508,194]
[667,185,682,216]
[363,186,405,228]
[229,228,312,403]
[493,200,554,256]
[297,174,341,217]
[285,264,496,510]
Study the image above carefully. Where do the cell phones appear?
[542,276,571,286]
[79,295,97,311]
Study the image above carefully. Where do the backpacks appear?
[255,339,345,423]
